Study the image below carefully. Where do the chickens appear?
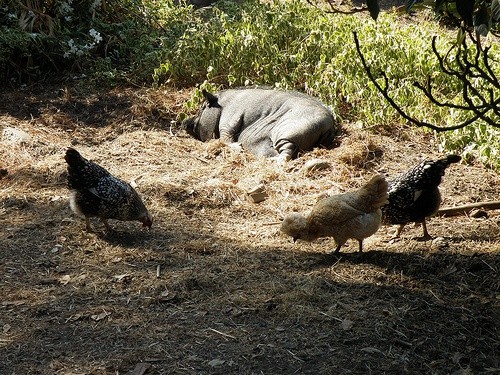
[282,154,461,256]
[64,148,153,235]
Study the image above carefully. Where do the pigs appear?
[191,87,337,163]
